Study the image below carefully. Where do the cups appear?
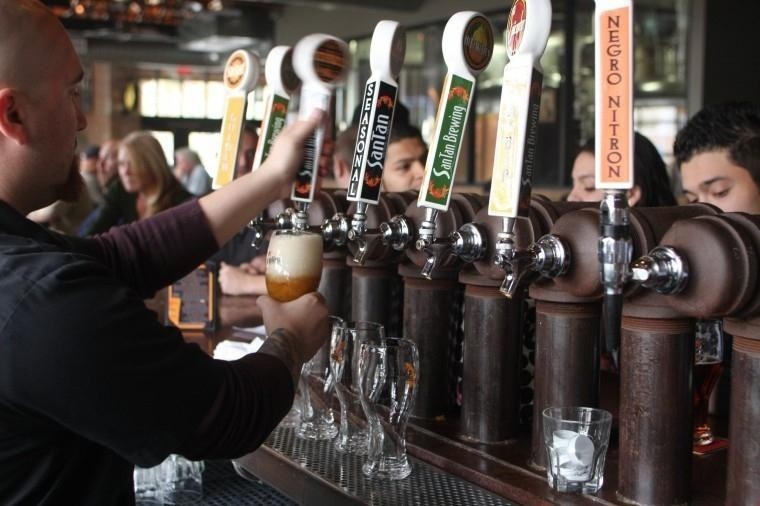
[133,451,206,506]
[357,336,421,482]
[265,230,326,303]
[330,319,387,457]
[539,404,614,493]
[295,314,351,442]
[278,380,304,431]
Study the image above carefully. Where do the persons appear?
[0,0,332,506]
[232,99,429,193]
[672,102,760,216]
[24,129,211,235]
[567,130,680,207]
